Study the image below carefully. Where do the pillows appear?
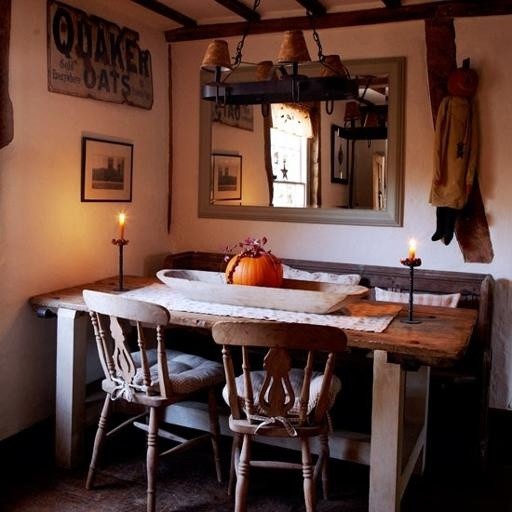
[372,285,460,309]
[281,263,362,286]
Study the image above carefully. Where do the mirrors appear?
[197,55,409,228]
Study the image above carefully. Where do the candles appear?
[118,213,127,239]
[409,240,416,260]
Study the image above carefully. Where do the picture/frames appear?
[80,137,135,203]
[210,151,244,201]
[329,123,350,186]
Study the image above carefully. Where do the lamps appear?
[339,80,388,148]
[199,1,359,115]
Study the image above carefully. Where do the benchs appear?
[163,250,496,469]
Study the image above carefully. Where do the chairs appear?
[80,287,227,511]
[209,318,348,511]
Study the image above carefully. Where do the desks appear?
[29,275,479,511]
